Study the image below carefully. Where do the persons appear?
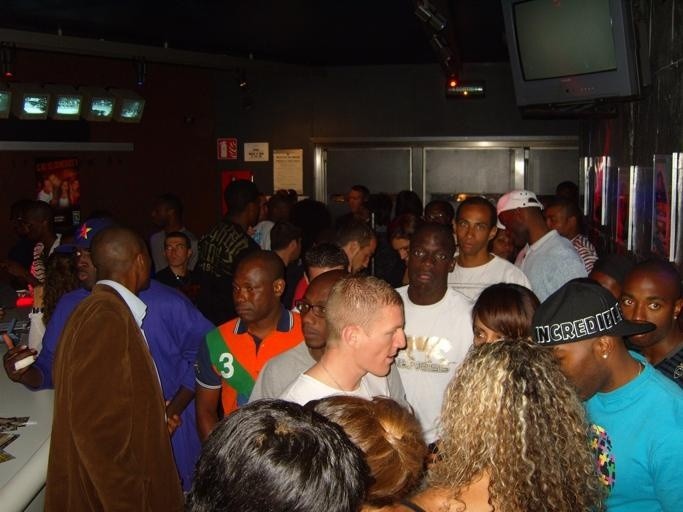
[0,178,683,512]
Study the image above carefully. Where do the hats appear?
[531,277,657,346]
[73,216,120,249]
[496,190,544,230]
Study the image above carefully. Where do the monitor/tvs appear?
[500,0,653,110]
[0,84,146,124]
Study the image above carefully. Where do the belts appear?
[33,307,46,313]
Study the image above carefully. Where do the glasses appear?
[294,299,327,318]
